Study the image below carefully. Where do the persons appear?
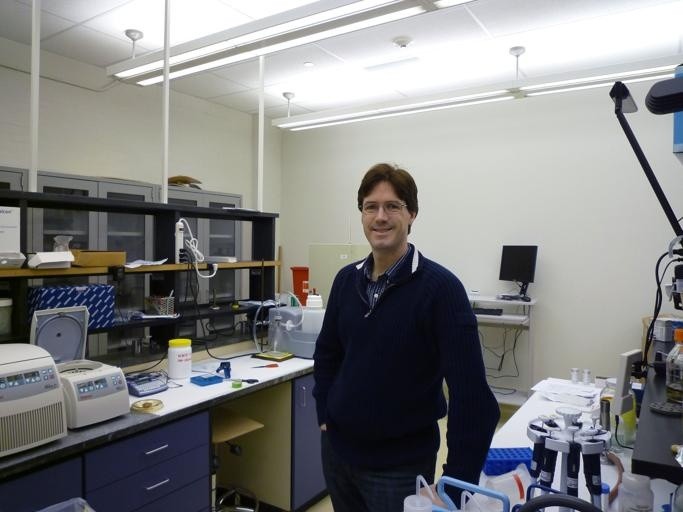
[309,163,501,512]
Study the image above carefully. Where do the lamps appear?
[106,0,476,88]
[270,46,683,131]
[610,80,683,246]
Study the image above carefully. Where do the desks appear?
[480,378,678,511]
[468,295,536,398]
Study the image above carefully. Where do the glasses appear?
[360,202,405,216]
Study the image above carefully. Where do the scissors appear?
[224,379,259,384]
[252,364,279,368]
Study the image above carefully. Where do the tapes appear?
[232,382,242,388]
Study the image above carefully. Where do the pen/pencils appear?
[169,289,174,297]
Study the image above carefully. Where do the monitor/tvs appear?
[497,245,538,300]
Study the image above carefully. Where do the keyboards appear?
[472,307,502,316]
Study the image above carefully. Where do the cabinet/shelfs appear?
[309,244,373,307]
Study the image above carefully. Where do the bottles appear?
[167,338,192,378]
[619,473,655,512]
[582,370,591,391]
[570,368,580,386]
[600,378,637,452]
[665,328,683,405]
[600,483,610,510]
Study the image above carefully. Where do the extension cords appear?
[175,221,185,265]
